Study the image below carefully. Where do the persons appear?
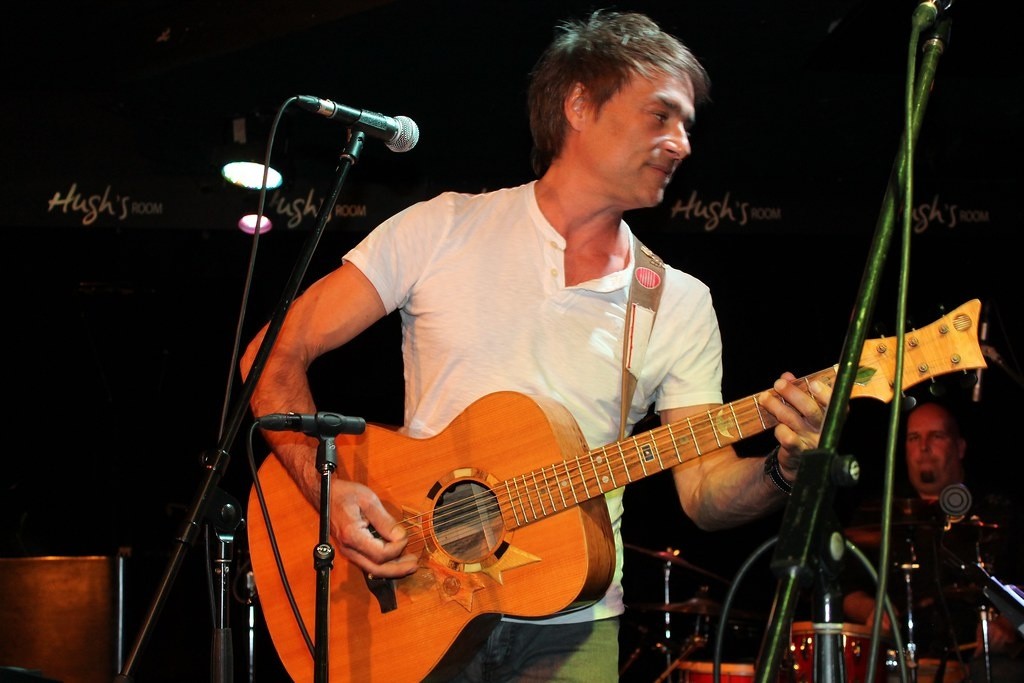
[840,401,1023,683]
[237,6,833,681]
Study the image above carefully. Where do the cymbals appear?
[623,541,733,586]
[654,595,744,626]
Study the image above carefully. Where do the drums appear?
[674,653,762,683]
[773,618,890,683]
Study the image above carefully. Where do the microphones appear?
[296,95,420,152]
[972,301,992,402]
[970,562,1024,634]
[912,0,953,31]
[255,413,366,434]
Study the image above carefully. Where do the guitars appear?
[211,290,999,683]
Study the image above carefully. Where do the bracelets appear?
[762,442,797,496]
